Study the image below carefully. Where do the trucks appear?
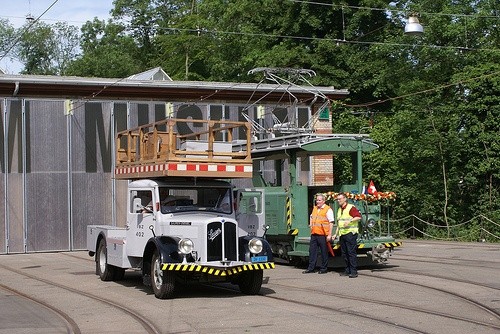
[85,177,276,300]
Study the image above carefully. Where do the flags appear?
[368,181,380,197]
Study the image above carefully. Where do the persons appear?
[219,189,243,213]
[302,195,335,274]
[331,193,362,277]
[142,189,176,214]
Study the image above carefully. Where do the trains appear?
[232,134,403,269]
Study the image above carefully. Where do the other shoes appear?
[302,270,315,274]
[339,271,351,276]
[318,270,328,274]
[349,272,358,278]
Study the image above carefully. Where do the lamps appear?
[405,14,424,36]
